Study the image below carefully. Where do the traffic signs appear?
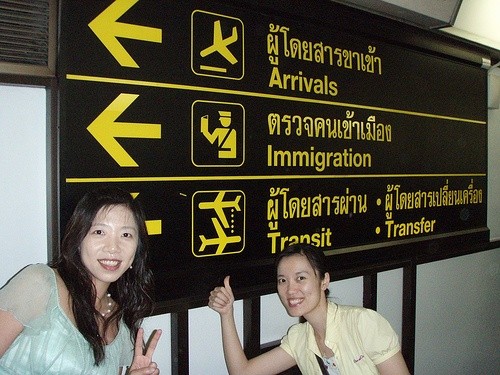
[47,0,492,316]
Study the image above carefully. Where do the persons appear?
[205,243,410,375]
[1,186,162,375]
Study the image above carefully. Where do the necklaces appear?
[320,348,328,362]
[100,291,111,317]
